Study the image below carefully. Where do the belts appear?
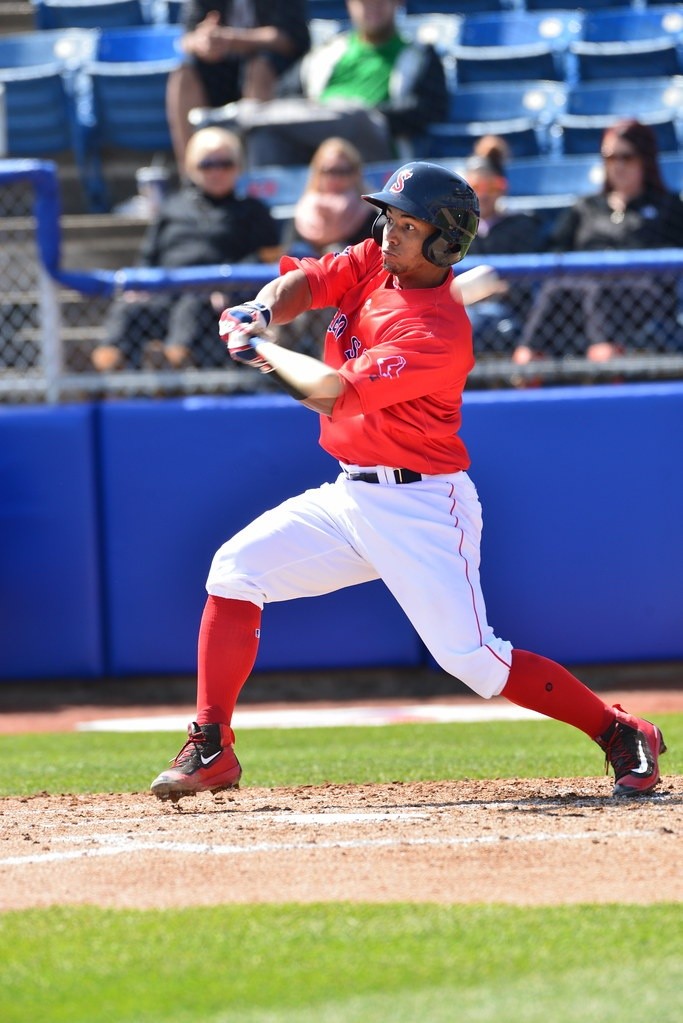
[344,468,423,484]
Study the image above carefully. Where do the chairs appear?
[0,0,683,351]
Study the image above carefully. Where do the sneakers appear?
[150,722,242,797]
[593,704,666,795]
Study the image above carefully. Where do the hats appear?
[469,135,508,169]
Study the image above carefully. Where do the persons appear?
[89,126,286,375]
[149,161,668,799]
[165,0,451,173]
[510,120,683,390]
[237,136,387,391]
[451,135,544,390]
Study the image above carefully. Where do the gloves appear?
[218,299,272,345]
[227,320,275,373]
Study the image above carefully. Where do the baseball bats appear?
[248,335,341,399]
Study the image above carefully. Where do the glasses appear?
[199,159,234,169]
[320,166,355,176]
[603,153,639,164]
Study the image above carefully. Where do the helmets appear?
[361,161,480,268]
